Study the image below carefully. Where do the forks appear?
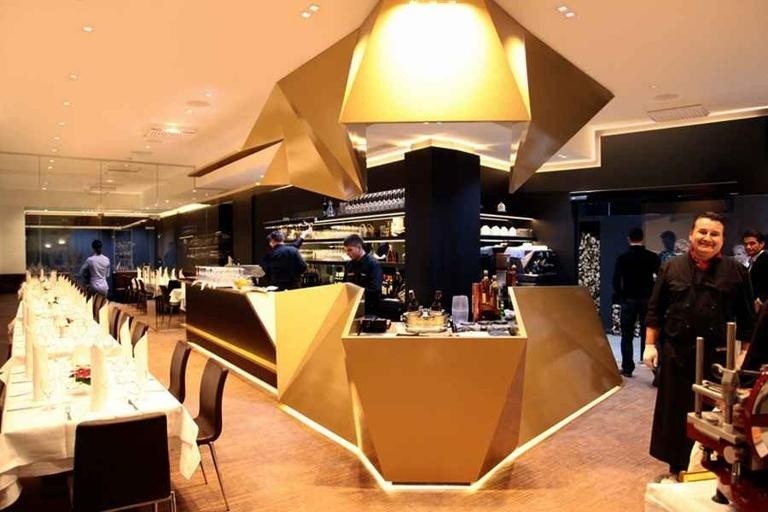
[66,405,71,420]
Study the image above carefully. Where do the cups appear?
[451,295,468,332]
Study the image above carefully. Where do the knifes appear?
[6,371,43,412]
[128,400,137,410]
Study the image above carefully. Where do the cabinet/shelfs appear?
[263,208,407,245]
[478,212,535,246]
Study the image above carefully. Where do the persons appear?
[643,211,755,475]
[164,242,176,267]
[79,240,111,298]
[261,230,311,285]
[270,231,307,290]
[612,226,768,384]
[342,234,382,315]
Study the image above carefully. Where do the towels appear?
[20,271,52,401]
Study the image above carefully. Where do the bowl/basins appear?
[399,309,449,332]
[357,319,388,334]
[479,223,516,236]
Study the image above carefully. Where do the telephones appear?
[359,314,387,333]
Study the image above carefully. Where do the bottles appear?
[406,290,444,316]
[471,269,507,331]
[381,274,393,297]
[195,266,244,288]
[730,436,750,484]
[303,264,319,285]
[323,188,405,260]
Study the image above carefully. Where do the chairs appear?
[53,274,149,409]
[161,339,193,403]
[180,357,231,510]
[66,411,177,510]
[115,266,190,328]
